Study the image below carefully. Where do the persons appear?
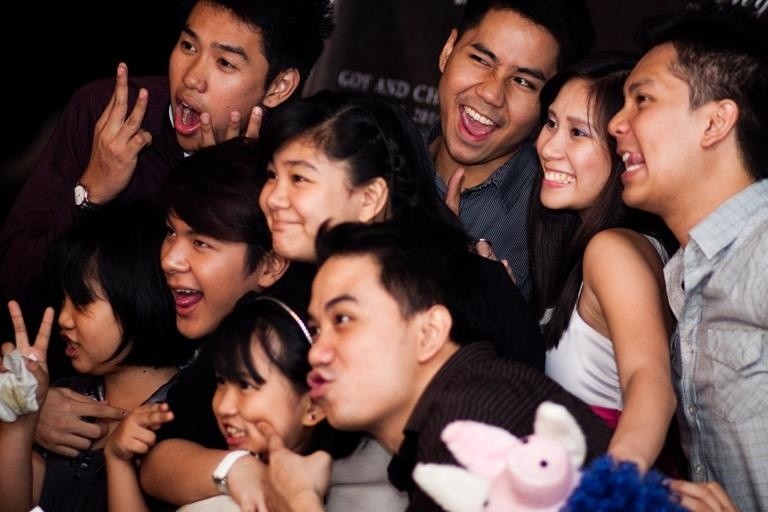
[0,0,768,512]
